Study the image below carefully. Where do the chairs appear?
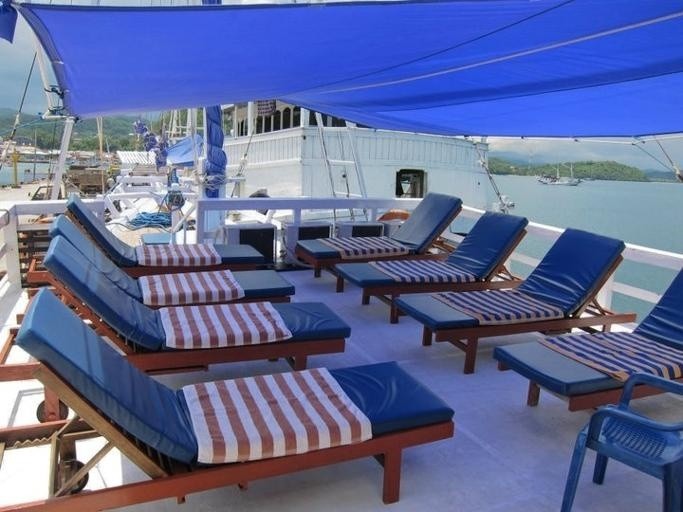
[294,192,464,278]
[13,213,299,308]
[394,225,642,378]
[335,210,531,328]
[557,369,683,512]
[63,189,264,272]
[489,264,681,410]
[3,233,352,371]
[0,285,455,511]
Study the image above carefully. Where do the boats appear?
[536,162,584,185]
